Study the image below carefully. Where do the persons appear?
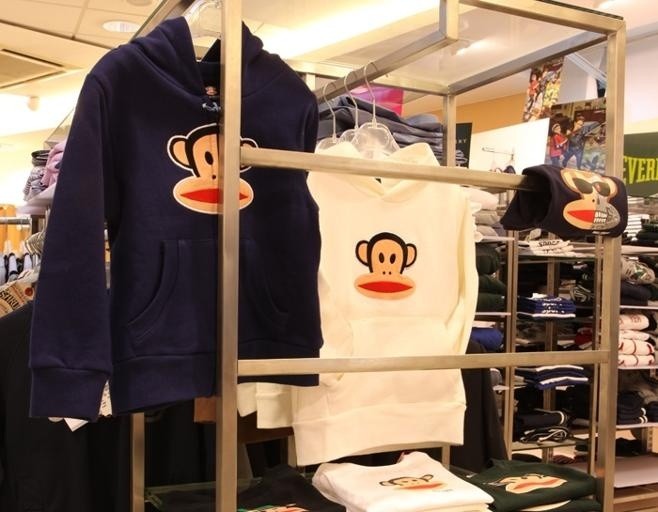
[562,114,586,170]
[550,123,567,167]
[528,60,552,113]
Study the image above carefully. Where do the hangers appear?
[0,212,50,284]
[180,2,243,63]
[296,63,468,205]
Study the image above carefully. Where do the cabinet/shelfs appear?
[509,245,658,505]
